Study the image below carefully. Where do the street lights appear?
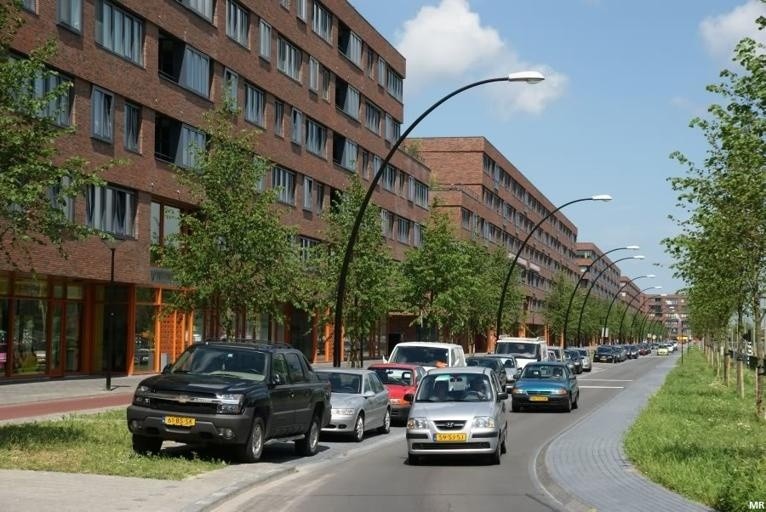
[561,242,639,345]
[601,274,657,343]
[331,69,546,366]
[619,275,679,346]
[575,254,648,344]
[493,191,614,347]
[99,232,121,393]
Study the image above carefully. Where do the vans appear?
[389,341,467,384]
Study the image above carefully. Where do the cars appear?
[493,352,521,392]
[645,343,685,357]
[363,364,422,418]
[315,362,390,439]
[512,364,579,413]
[547,346,647,371]
[464,357,506,386]
[406,367,505,462]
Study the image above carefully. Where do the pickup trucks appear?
[125,337,332,464]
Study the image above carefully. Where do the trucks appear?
[493,335,543,377]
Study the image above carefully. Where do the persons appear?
[462,380,487,400]
[551,368,560,379]
[428,382,455,402]
